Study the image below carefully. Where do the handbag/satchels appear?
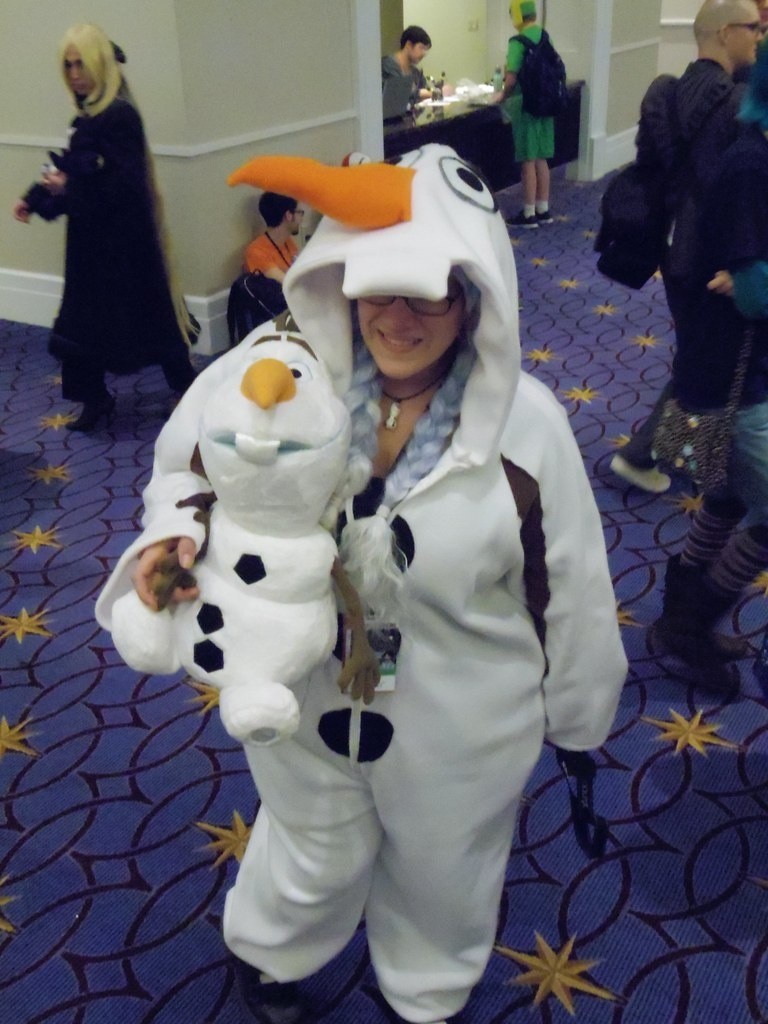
[651,400,736,486]
[594,166,674,289]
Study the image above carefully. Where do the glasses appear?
[289,210,304,216]
[728,22,760,31]
[359,290,461,317]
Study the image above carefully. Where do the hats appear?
[507,0,537,28]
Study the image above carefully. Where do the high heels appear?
[65,384,115,430]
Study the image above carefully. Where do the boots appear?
[645,556,748,698]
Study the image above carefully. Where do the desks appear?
[383,78,584,197]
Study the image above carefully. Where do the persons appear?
[486,0,555,229]
[243,190,307,284]
[92,138,631,1024]
[380,24,433,125]
[11,22,198,433]
[608,0,768,700]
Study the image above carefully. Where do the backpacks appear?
[227,268,288,347]
[509,28,566,118]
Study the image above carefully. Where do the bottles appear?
[494,68,503,92]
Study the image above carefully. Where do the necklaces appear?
[376,364,447,430]
[265,232,293,268]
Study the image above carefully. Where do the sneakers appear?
[535,210,553,224]
[506,212,539,229]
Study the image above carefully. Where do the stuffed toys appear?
[107,330,382,744]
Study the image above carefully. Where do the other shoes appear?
[610,451,671,494]
[235,959,301,1024]
